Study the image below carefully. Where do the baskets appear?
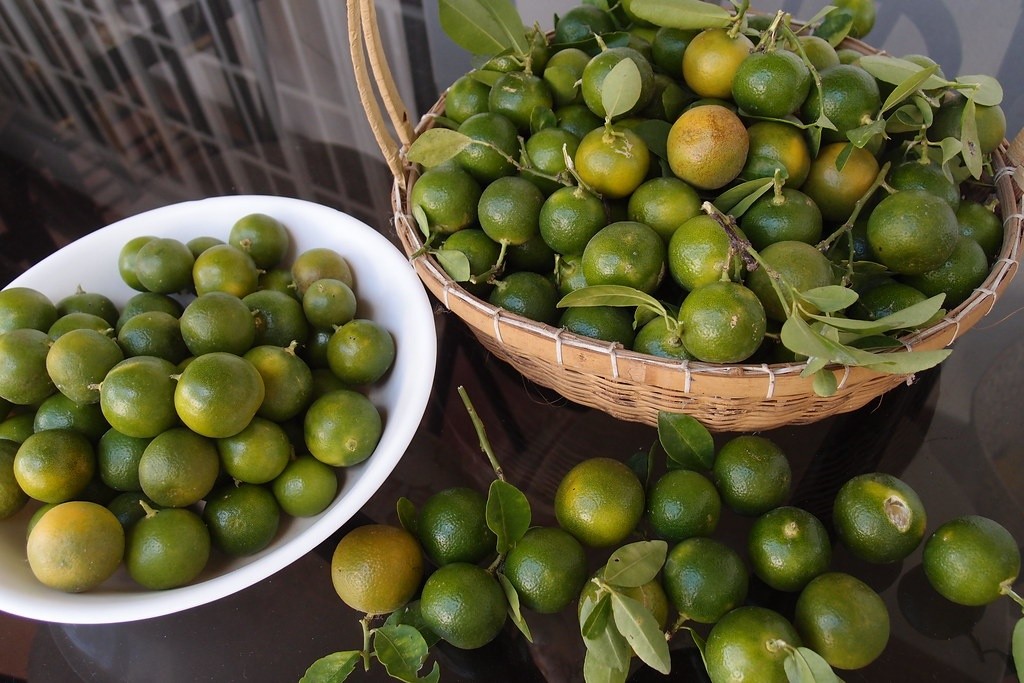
[346,0,1024,432]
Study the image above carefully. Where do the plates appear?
[0,196,437,626]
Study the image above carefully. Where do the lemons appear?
[0,214,395,595]
[412,8,1007,365]
[330,435,1021,683]
